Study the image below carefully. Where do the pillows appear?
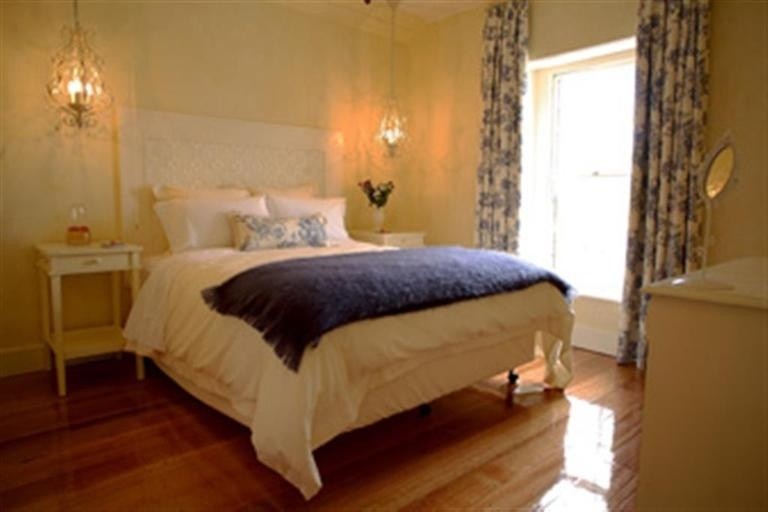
[151,184,351,254]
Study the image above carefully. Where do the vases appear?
[372,207,385,233]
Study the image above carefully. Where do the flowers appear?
[357,179,397,207]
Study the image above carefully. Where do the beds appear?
[115,108,574,504]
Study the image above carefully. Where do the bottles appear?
[65,207,92,247]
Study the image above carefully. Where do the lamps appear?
[46,1,115,133]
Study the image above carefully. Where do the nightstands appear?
[33,240,143,397]
[349,229,426,247]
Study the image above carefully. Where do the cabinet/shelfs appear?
[635,256,767,512]
